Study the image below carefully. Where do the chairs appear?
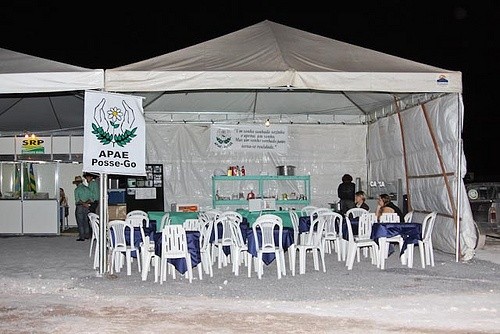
[87,203,438,286]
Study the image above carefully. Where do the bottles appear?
[490,206,496,223]
[227,165,247,176]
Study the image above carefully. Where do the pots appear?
[277,165,295,175]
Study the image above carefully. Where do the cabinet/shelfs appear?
[211,174,310,228]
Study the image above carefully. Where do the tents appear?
[0,46,104,276]
[103,20,478,274]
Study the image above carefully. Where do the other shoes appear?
[76,238,85,241]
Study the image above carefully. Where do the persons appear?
[374,194,405,258]
[58,188,69,222]
[82,172,100,239]
[73,175,95,242]
[347,190,369,220]
[338,174,356,220]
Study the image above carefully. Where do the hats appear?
[73,176,83,183]
